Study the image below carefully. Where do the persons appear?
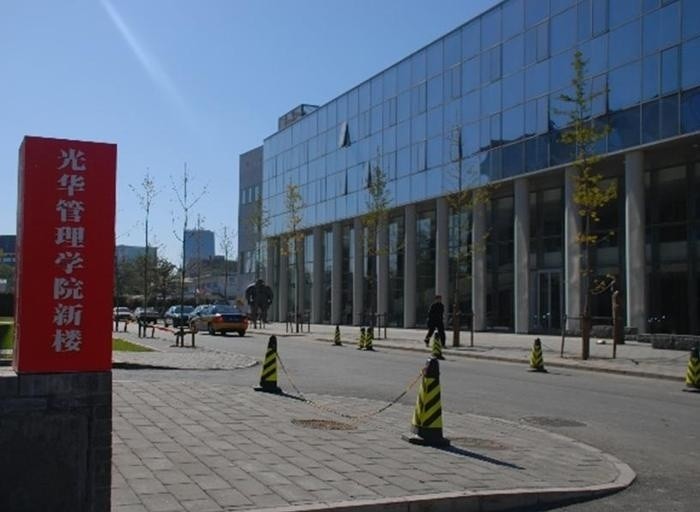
[424,295,446,348]
[245,279,274,324]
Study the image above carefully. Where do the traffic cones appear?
[526,338,546,374]
[251,333,285,392]
[357,327,374,352]
[404,355,453,447]
[680,344,699,393]
[430,333,446,360]
[330,324,342,347]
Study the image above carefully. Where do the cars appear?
[163,304,194,328]
[189,303,209,318]
[189,305,247,338]
[288,310,295,321]
[304,308,310,321]
[113,306,132,322]
[134,307,161,324]
